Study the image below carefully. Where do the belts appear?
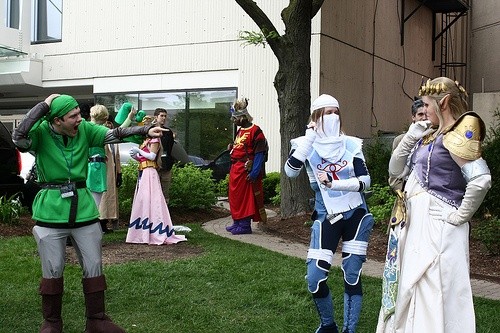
[42,181,86,190]
[89,157,105,162]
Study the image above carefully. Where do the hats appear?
[309,93,340,115]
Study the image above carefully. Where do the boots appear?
[38,278,66,333]
[81,274,125,333]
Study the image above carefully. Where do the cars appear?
[188,149,232,184]
[0,121,24,201]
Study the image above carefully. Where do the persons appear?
[126,115,164,245]
[225,97,269,235]
[283,94,372,332]
[373,77,491,333]
[10,93,170,333]
[84,104,135,234]
[147,108,175,205]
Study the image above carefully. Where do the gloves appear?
[429,199,456,222]
[129,151,136,159]
[131,147,139,154]
[408,120,436,142]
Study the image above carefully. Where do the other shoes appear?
[315,322,338,333]
[226,221,252,234]
